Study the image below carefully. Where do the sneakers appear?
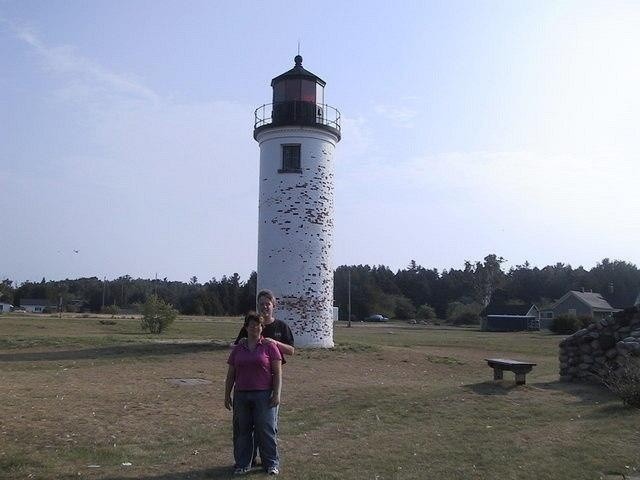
[233,466,251,474]
[266,467,279,475]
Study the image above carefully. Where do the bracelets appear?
[276,340,280,348]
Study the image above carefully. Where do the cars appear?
[364,315,388,322]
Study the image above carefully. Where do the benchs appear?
[484,358,537,385]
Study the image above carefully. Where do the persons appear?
[232,288,296,470]
[224,310,284,476]
